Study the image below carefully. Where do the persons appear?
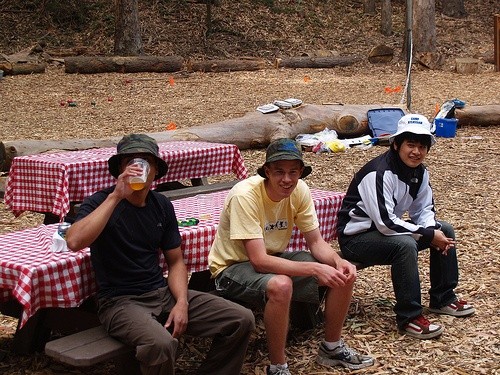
[66,135,255,375]
[207,139,375,375]
[336,113,475,340]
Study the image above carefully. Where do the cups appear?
[127,158,150,190]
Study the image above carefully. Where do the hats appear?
[389,114,437,146]
[257,138,312,179]
[108,133,168,181]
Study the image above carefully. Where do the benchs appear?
[156,179,237,201]
[44,252,373,375]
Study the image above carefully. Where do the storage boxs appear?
[434,118,458,138]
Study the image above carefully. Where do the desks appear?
[3,141,249,225]
[0,189,346,355]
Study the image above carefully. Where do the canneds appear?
[58,223,71,240]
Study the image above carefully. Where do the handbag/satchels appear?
[367,108,406,145]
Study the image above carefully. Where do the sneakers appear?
[265,364,293,375]
[429,297,475,316]
[315,341,376,369]
[398,313,444,339]
